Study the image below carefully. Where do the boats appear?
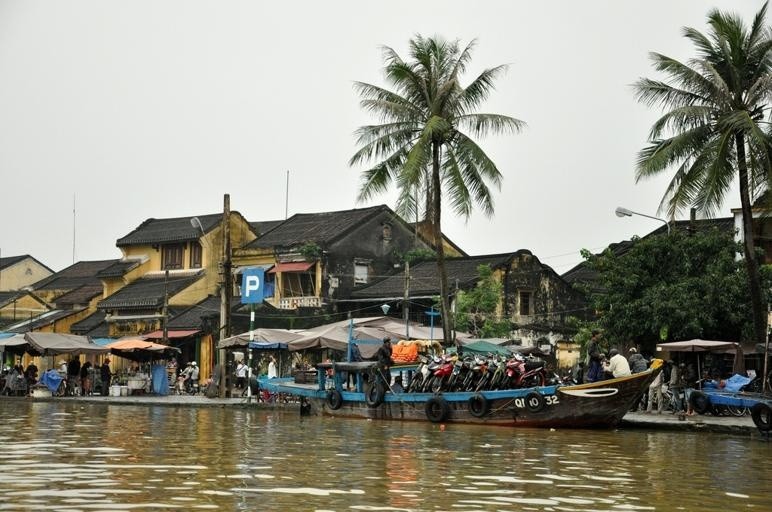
[257,308,663,428]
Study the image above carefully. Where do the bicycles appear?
[651,371,772,416]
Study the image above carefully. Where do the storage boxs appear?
[128,380,146,390]
[294,370,318,384]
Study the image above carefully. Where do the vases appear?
[108,384,132,397]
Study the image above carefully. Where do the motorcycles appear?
[177,369,198,396]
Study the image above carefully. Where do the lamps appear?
[151,243,159,253]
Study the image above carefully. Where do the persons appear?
[268,354,279,380]
[390,375,406,392]
[570,329,698,417]
[376,334,394,392]
[236,359,249,388]
[360,373,374,393]
[0,357,200,398]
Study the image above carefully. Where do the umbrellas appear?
[655,337,738,378]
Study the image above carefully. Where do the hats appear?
[592,329,601,334]
[105,359,110,362]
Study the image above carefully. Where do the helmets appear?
[629,348,638,353]
[187,362,197,366]
[383,337,390,343]
[609,349,618,356]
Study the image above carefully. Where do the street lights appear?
[615,206,670,237]
[190,217,210,247]
